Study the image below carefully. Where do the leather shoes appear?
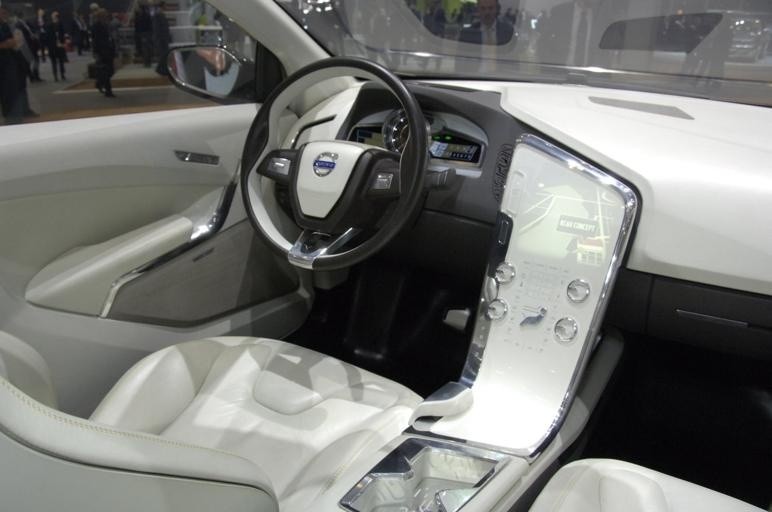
[96,82,116,98]
[23,110,41,117]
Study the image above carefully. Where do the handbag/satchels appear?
[88,40,113,79]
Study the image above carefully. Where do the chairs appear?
[1,328,424,511]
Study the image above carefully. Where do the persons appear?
[409,1,517,45]
[0,0,172,126]
